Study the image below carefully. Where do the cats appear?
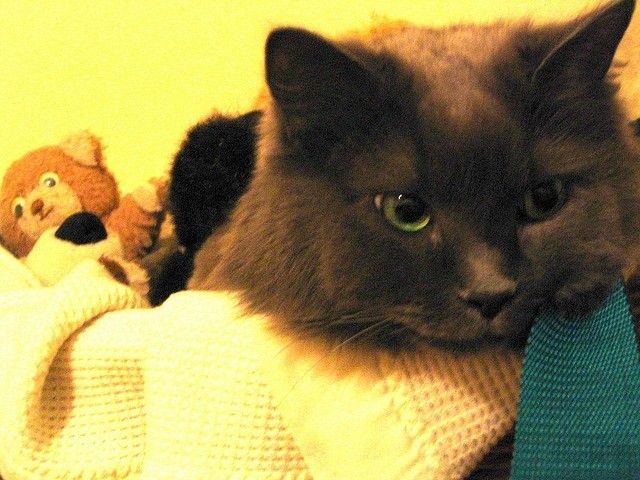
[141,0,640,360]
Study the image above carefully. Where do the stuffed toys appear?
[1,129,173,298]
